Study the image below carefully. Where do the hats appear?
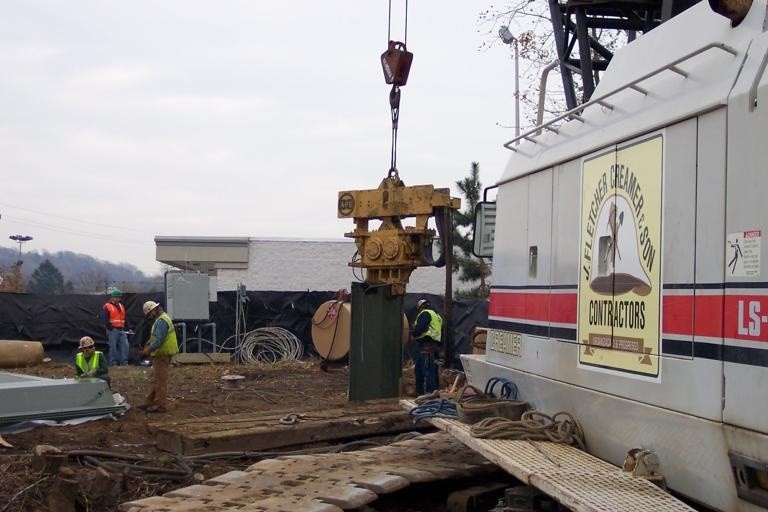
[110,289,123,298]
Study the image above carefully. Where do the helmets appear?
[77,335,95,350]
[415,299,431,312]
[142,300,161,316]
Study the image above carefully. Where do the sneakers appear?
[135,402,166,412]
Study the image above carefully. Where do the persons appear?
[102,290,133,366]
[74,336,110,387]
[141,301,179,413]
[410,300,443,396]
